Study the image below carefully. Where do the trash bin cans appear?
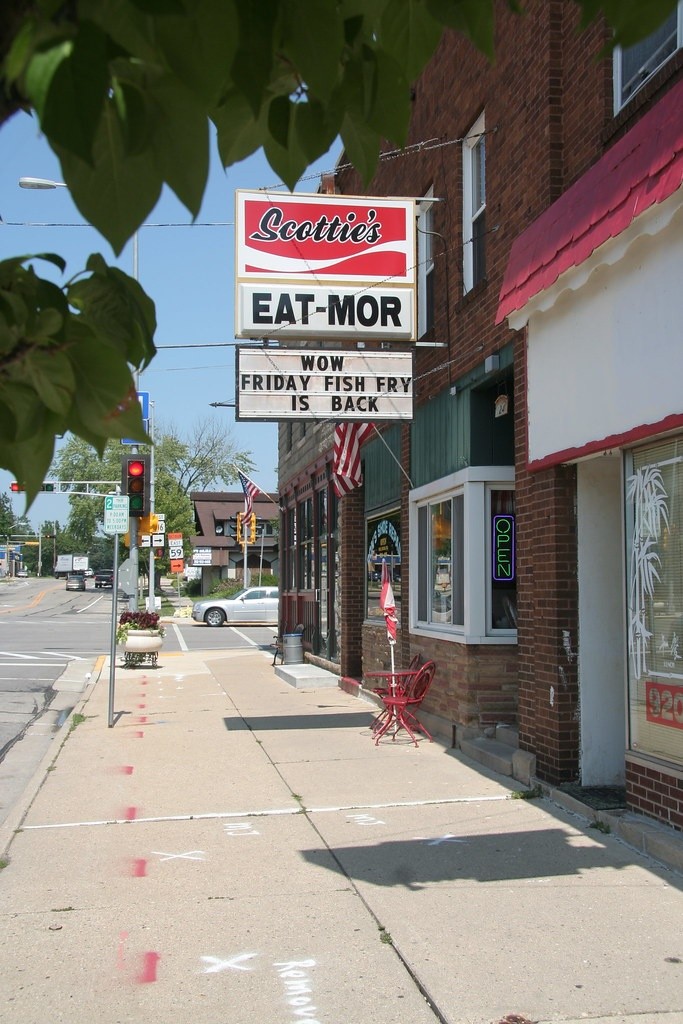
[36,573,40,577]
[129,596,138,612]
[283,634,305,664]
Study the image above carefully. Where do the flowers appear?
[115,609,167,669]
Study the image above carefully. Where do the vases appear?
[122,629,163,652]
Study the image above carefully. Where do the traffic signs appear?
[168,532,184,573]
[152,513,166,548]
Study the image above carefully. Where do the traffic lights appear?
[10,481,55,493]
[248,513,263,545]
[120,454,150,518]
[228,512,243,546]
[45,534,57,538]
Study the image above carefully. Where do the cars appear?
[65,574,87,591]
[85,568,94,578]
[190,585,279,628]
[15,569,28,578]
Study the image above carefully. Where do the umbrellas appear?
[379,557,399,735]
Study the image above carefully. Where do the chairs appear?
[371,654,436,748]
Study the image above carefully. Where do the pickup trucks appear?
[94,568,114,589]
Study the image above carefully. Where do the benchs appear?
[269,623,306,666]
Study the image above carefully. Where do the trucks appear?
[53,554,91,580]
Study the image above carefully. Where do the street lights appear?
[15,173,139,615]
[6,522,28,578]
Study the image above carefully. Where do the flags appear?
[239,472,260,526]
[331,422,375,499]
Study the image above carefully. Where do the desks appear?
[365,668,420,739]
